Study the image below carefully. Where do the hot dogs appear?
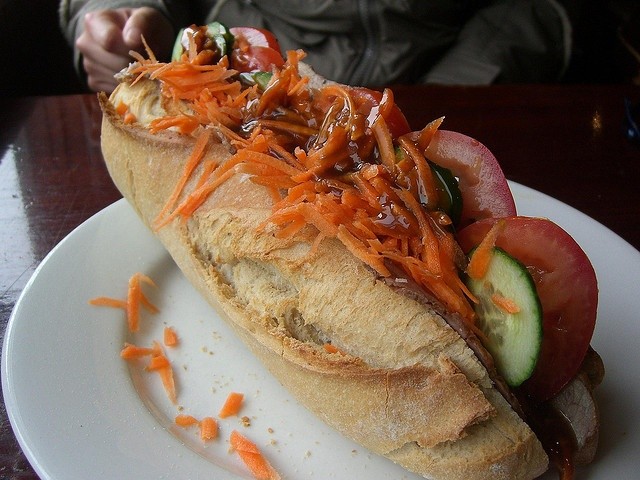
[97,22,605,480]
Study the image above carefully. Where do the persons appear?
[58,1,572,94]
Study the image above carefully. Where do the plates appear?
[1,177,640,480]
